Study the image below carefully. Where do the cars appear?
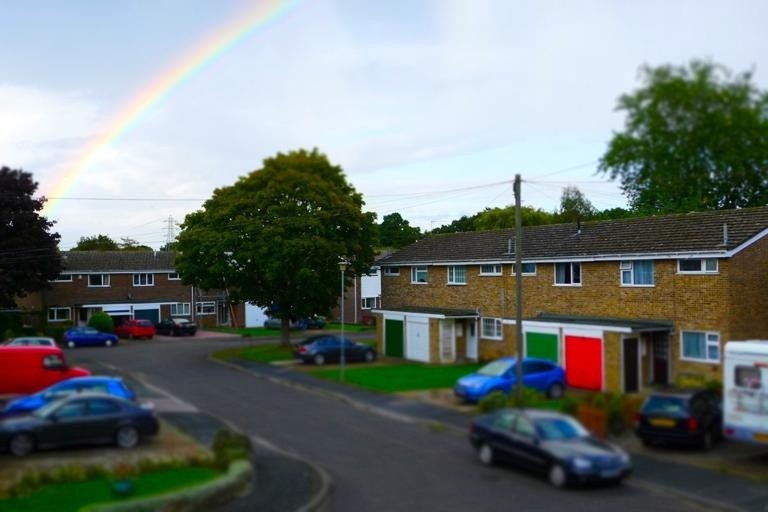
[466,407,635,485]
[1,391,161,459]
[113,319,156,340]
[1,335,57,347]
[1,374,138,416]
[452,356,568,403]
[155,317,197,337]
[633,386,722,450]
[306,313,328,330]
[264,315,307,332]
[292,332,379,366]
[62,324,119,349]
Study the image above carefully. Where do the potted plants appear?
[576,392,611,440]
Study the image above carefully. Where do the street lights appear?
[337,261,349,367]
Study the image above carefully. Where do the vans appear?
[720,338,767,447]
[0,344,91,402]
